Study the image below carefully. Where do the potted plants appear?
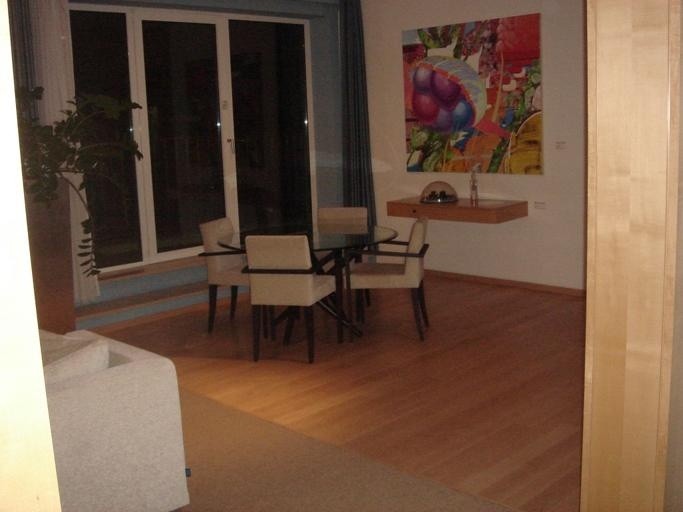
[19,86,144,334]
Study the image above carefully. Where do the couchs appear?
[38,327,191,512]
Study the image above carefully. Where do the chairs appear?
[317,205,370,321]
[241,235,338,363]
[197,217,250,334]
[344,217,428,341]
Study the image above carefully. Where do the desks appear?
[218,225,398,336]
